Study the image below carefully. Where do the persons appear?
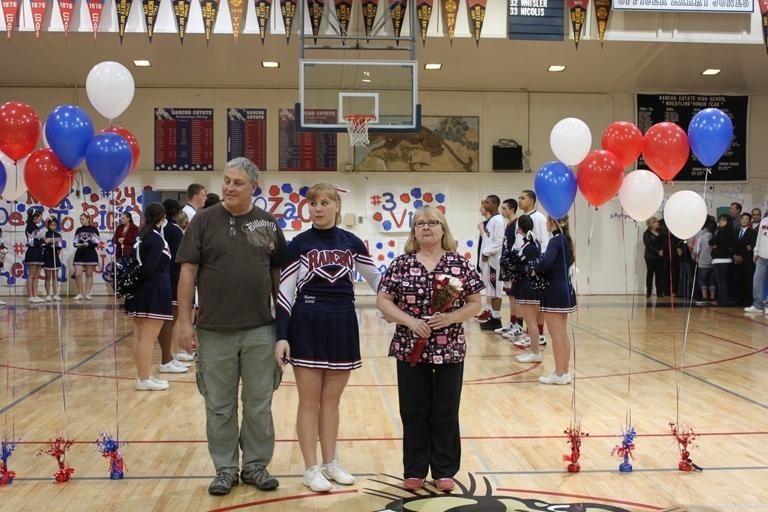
[23,208,45,303]
[274,182,397,492]
[477,190,579,385]
[0,228,8,305]
[112,184,222,391]
[175,157,287,495]
[643,203,768,314]
[73,213,100,300]
[376,206,486,492]
[42,216,62,301]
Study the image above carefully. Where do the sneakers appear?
[709,299,717,304]
[53,295,62,301]
[240,466,279,490]
[73,295,84,300]
[539,372,572,385]
[160,358,191,373]
[46,296,52,302]
[135,376,169,391]
[435,478,456,492]
[209,471,239,495]
[494,322,514,334]
[85,294,92,300]
[502,323,523,338]
[480,316,502,329]
[176,351,196,361]
[474,309,492,323]
[404,478,424,491]
[303,465,333,492]
[514,335,546,346]
[696,297,710,304]
[321,459,355,485]
[744,305,764,313]
[28,296,45,303]
[516,351,542,363]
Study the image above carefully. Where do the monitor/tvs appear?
[493,145,522,170]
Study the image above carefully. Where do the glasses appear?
[414,219,442,228]
[229,218,238,240]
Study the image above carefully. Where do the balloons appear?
[534,108,735,241]
[0,61,140,208]
